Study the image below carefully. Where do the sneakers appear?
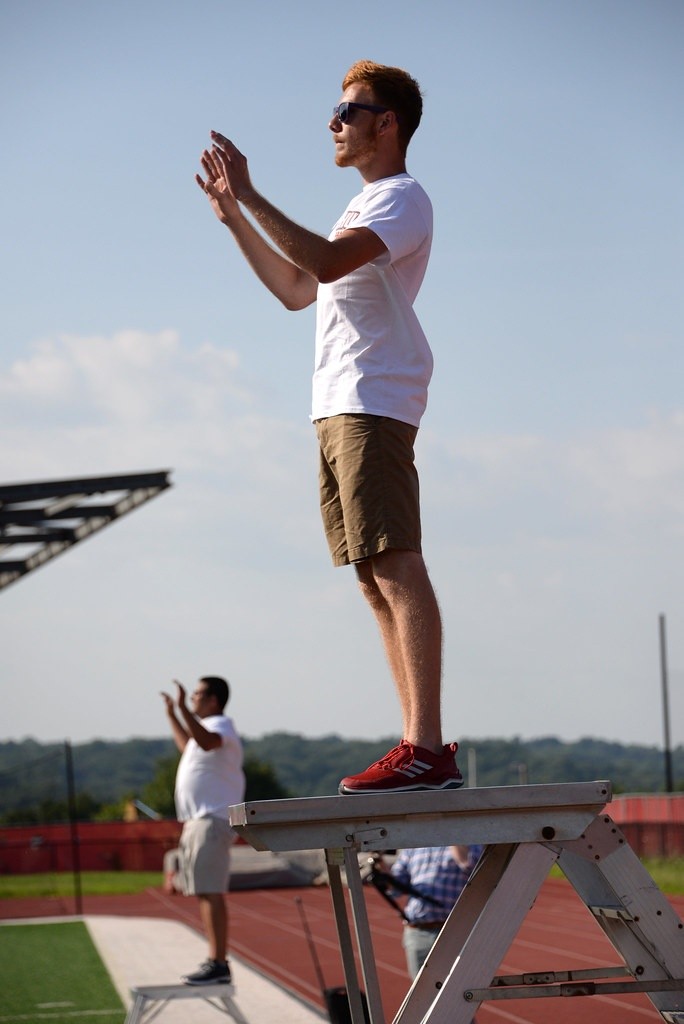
[180,956,232,986]
[337,738,465,795]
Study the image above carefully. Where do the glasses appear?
[332,102,388,123]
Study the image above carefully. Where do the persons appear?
[195,60,466,793]
[368,843,485,985]
[160,677,245,988]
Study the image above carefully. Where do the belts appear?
[408,921,446,929]
